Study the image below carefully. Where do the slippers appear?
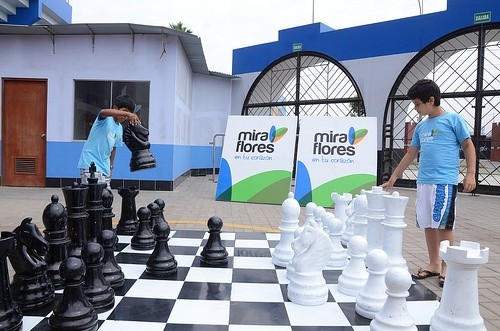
[412,267,440,279]
[439,275,445,287]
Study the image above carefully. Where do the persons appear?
[77,93,143,199]
[376,78,476,288]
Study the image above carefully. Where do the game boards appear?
[12,226,442,330]
